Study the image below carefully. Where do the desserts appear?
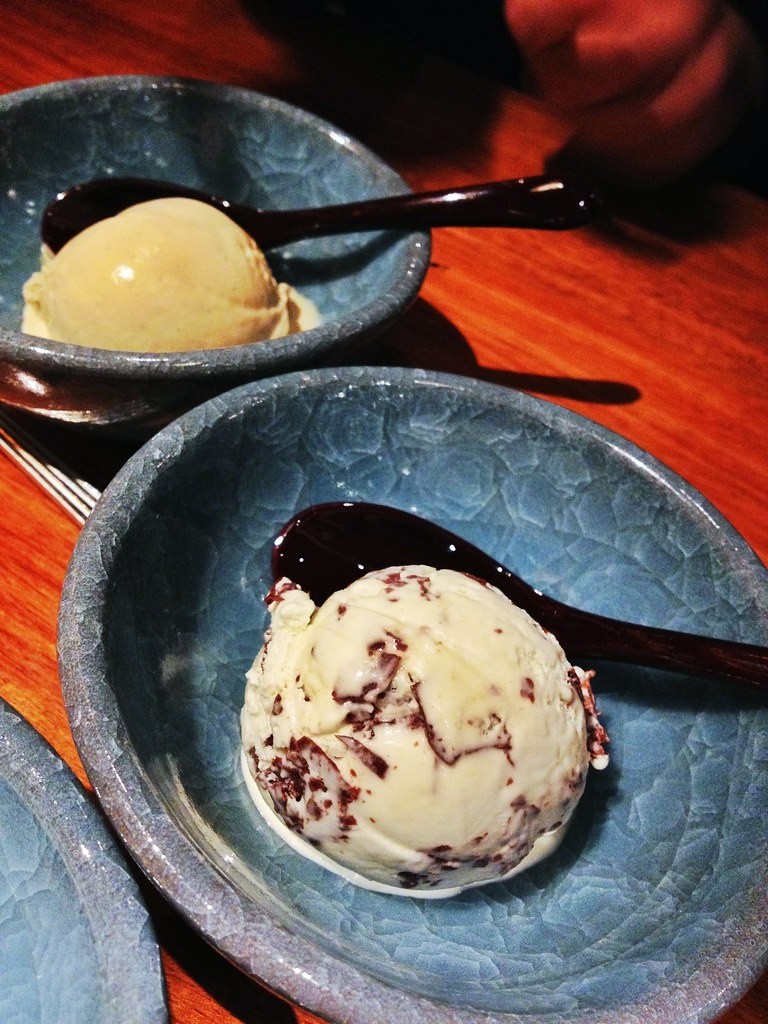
[22,196,320,353]
[238,561,610,899]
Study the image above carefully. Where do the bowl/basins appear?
[0,699,176,1024]
[54,366,767,1024]
[0,74,433,446]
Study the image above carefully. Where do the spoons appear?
[40,175,598,268]
[269,500,767,706]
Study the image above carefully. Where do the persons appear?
[504,0,768,207]
